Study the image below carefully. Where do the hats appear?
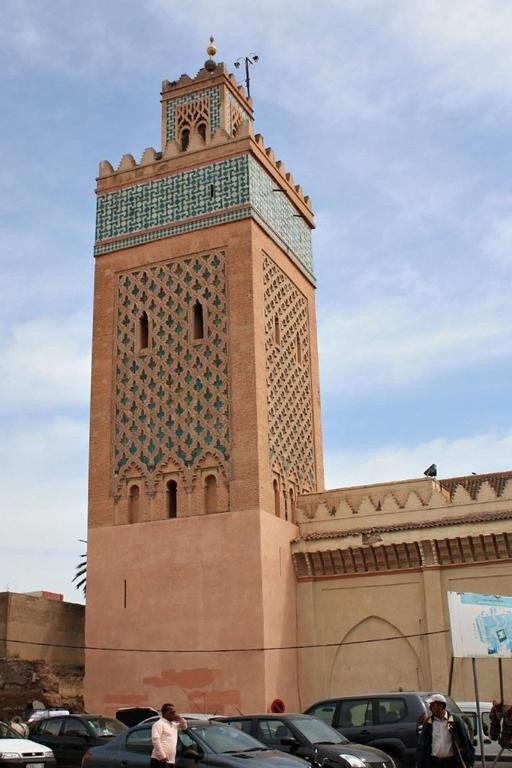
[425,693,447,704]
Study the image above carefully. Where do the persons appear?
[414,694,474,768]
[150,703,187,768]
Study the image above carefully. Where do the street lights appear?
[234,53,259,97]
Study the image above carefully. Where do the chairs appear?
[275,725,290,739]
[339,706,405,727]
